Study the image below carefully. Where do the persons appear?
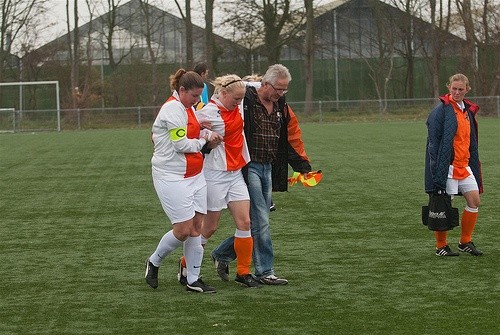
[424,74,485,257]
[143,63,312,293]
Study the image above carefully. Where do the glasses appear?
[266,83,289,94]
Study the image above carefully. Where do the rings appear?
[207,133,208,135]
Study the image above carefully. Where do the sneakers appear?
[178,255,188,285]
[458,241,482,256]
[184,280,216,295]
[211,252,230,282]
[234,273,264,288]
[435,244,459,257]
[145,258,159,288]
[260,274,288,285]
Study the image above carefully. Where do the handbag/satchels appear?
[427,188,453,231]
